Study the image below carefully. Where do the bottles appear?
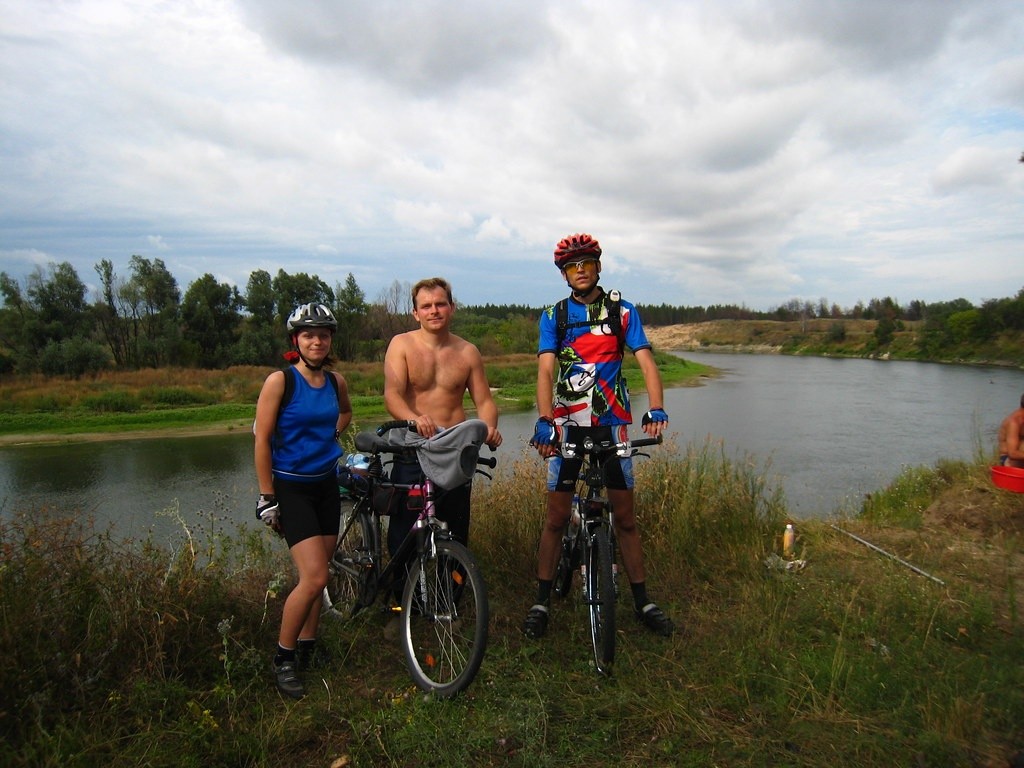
[566,494,580,539]
[346,453,369,466]
[782,524,794,558]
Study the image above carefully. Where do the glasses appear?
[563,258,596,272]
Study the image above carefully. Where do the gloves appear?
[256,494,280,530]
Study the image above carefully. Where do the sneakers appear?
[633,599,675,636]
[521,602,548,638]
[383,616,400,640]
[272,654,306,698]
[299,647,331,668]
[440,619,462,634]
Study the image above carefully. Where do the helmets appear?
[554,233,602,270]
[287,303,337,334]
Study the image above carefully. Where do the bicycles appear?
[528,428,663,676]
[320,419,498,698]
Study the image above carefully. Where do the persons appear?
[522,234,682,637]
[254,303,353,700]
[384,277,502,641]
[998,394,1024,469]
